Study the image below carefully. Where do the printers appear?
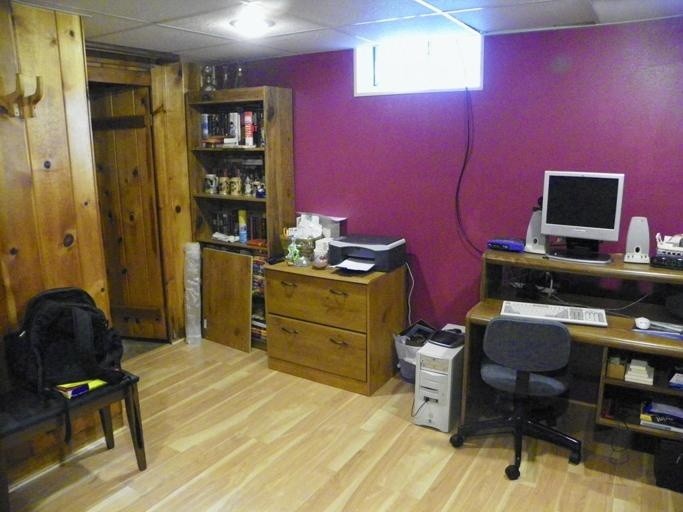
[329,234,408,272]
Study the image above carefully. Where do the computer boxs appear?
[414,323,466,433]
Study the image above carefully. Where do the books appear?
[54,378,107,400]
[202,205,267,343]
[623,356,682,434]
[200,109,268,148]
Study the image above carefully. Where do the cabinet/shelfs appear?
[185,84,295,350]
[263,261,410,396]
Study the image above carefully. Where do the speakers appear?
[624,216,651,264]
[523,210,547,255]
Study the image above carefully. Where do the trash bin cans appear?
[393,323,436,383]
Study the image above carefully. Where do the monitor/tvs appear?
[540,170,625,264]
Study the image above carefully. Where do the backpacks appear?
[9,296,124,400]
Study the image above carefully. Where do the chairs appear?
[448,317,581,480]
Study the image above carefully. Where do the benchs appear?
[2,285,146,512]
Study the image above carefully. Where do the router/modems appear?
[488,238,523,251]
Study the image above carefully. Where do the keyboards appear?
[499,300,608,327]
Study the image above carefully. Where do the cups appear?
[204,174,265,197]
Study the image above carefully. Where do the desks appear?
[459,247,682,442]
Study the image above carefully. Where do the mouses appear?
[635,317,650,329]
